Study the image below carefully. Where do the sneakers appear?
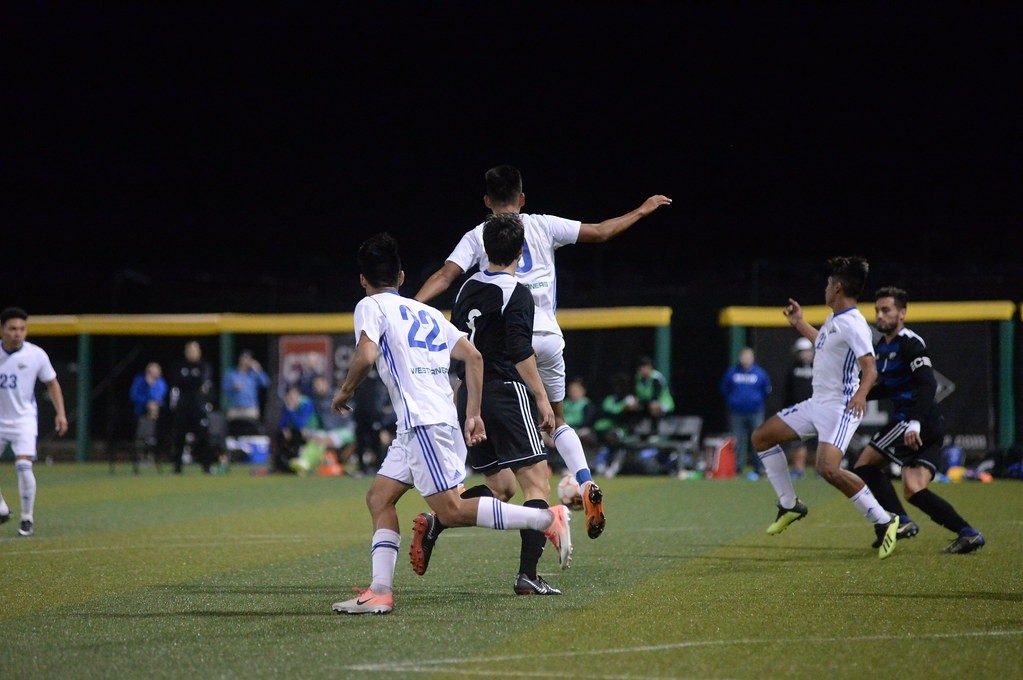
[409,512,438,576]
[766,497,808,535]
[872,511,900,559]
[939,531,987,555]
[19,521,34,536]
[872,520,918,547]
[581,481,606,540]
[512,574,561,595]
[331,587,394,613]
[545,505,573,571]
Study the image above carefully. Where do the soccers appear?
[556,474,585,513]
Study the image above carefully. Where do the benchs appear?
[645,417,701,476]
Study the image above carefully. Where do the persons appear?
[632,357,676,461]
[721,347,772,478]
[408,211,561,595]
[0,306,69,537]
[413,163,673,541]
[772,336,813,480]
[592,374,649,480]
[161,341,215,476]
[545,377,594,476]
[331,234,573,614]
[853,285,986,555]
[751,255,901,559]
[131,361,169,476]
[267,341,395,476]
[220,350,273,459]
